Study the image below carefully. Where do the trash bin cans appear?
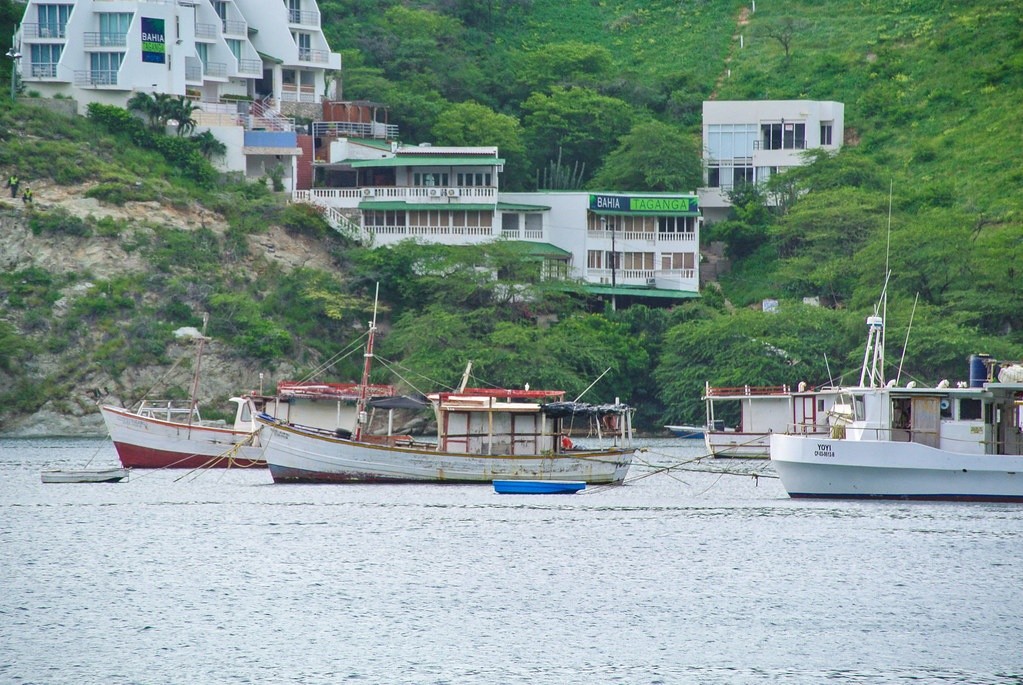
[970,354,993,388]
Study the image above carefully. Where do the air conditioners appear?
[447,188,460,198]
[646,278,656,285]
[428,188,441,197]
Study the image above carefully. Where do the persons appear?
[5,173,19,199]
[23,187,32,203]
[563,437,573,449]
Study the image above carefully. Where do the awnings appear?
[557,283,703,298]
[348,136,412,152]
[408,202,495,216]
[485,242,572,258]
[496,202,553,210]
[591,209,702,218]
[358,200,408,211]
[342,155,507,187]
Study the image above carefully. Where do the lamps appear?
[362,187,376,196]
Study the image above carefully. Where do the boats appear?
[662,424,738,439]
[39,467,132,486]
[254,278,639,486]
[766,428,1022,500]
[698,178,1022,459]
[492,478,588,495]
[83,310,268,471]
